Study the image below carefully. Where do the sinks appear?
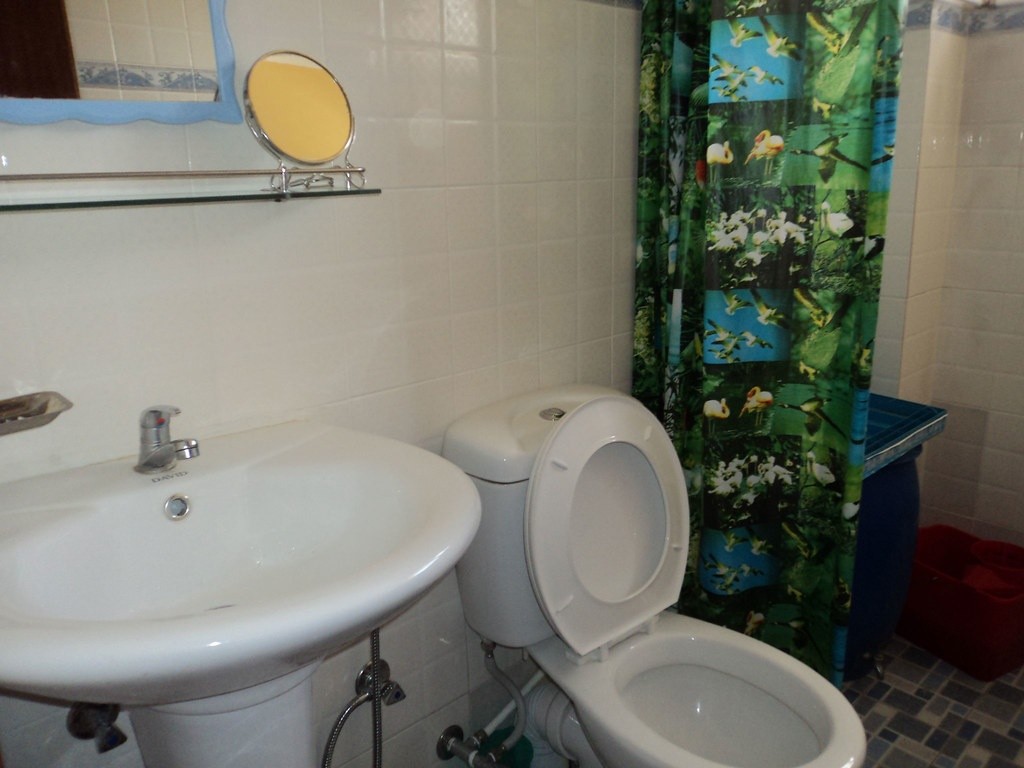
[2,419,484,705]
[861,389,949,482]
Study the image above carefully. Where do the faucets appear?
[134,401,201,474]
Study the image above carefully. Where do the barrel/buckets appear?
[842,445,922,680]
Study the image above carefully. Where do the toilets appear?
[443,383,866,768]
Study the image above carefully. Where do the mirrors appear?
[0,0,243,125]
